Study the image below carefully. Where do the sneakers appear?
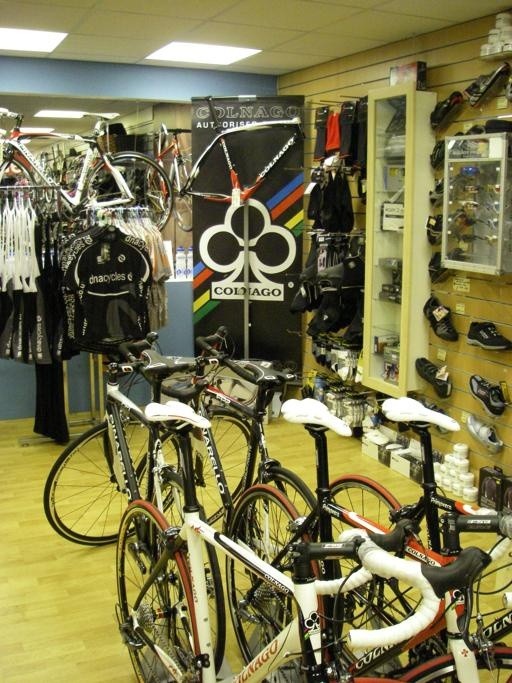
[428,90,464,132]
[462,63,510,108]
[462,319,511,353]
[467,374,508,419]
[421,296,461,344]
[425,135,461,288]
[414,357,457,399]
[464,412,505,453]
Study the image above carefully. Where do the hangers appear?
[1,184,152,227]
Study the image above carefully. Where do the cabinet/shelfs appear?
[361,81,436,403]
[440,131,511,277]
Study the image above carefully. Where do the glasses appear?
[452,162,511,253]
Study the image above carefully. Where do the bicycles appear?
[0,112,192,233]
